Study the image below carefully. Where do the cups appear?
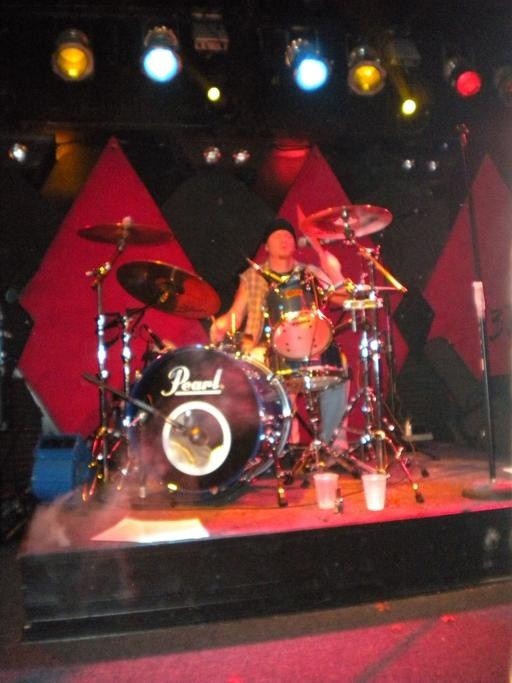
[361,473,390,513]
[313,470,339,509]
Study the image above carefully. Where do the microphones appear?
[183,423,208,447]
[298,237,338,248]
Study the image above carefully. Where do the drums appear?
[128,345,292,505]
[262,278,336,361]
[262,344,350,394]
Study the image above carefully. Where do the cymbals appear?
[301,203,392,241]
[116,261,221,318]
[75,224,173,245]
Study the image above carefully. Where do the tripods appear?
[116,321,151,512]
[283,295,430,503]
[81,287,120,506]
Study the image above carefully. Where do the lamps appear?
[48,20,512,123]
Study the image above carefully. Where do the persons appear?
[209,218,349,444]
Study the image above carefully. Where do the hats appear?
[263,218,295,243]
